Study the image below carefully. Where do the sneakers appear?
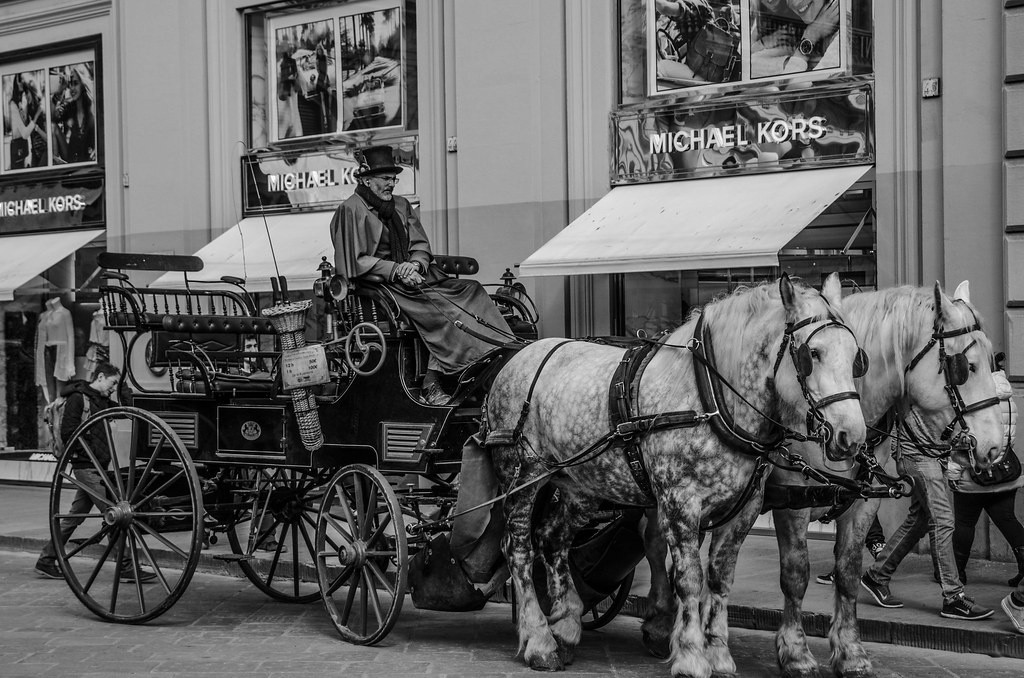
[861,574,903,607]
[940,596,995,619]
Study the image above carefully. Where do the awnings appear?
[518,163,873,276]
[0,228,106,301]
[150,205,419,291]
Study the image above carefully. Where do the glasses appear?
[370,176,399,184]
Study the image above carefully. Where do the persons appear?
[35,296,76,405]
[654,0,840,87]
[201,332,287,553]
[330,146,519,408]
[816,347,1024,633]
[8,63,96,169]
[85,296,110,383]
[35,364,157,582]
[282,44,337,116]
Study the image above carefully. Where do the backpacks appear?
[43,394,91,460]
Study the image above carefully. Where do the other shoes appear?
[256,539,286,554]
[419,381,450,407]
[1001,592,1024,634]
[34,562,64,578]
[120,566,155,583]
[816,571,836,584]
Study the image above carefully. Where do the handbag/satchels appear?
[970,444,1021,486]
[686,18,733,83]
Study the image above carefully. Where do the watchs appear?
[412,261,420,267]
[797,37,815,56]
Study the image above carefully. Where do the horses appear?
[486,271,866,678]
[641,280,1005,678]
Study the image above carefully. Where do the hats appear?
[353,145,404,178]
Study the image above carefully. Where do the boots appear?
[935,552,969,583]
[1007,545,1024,586]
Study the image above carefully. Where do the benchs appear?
[95,248,281,393]
[316,253,539,407]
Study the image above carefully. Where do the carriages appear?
[46,251,1007,677]
